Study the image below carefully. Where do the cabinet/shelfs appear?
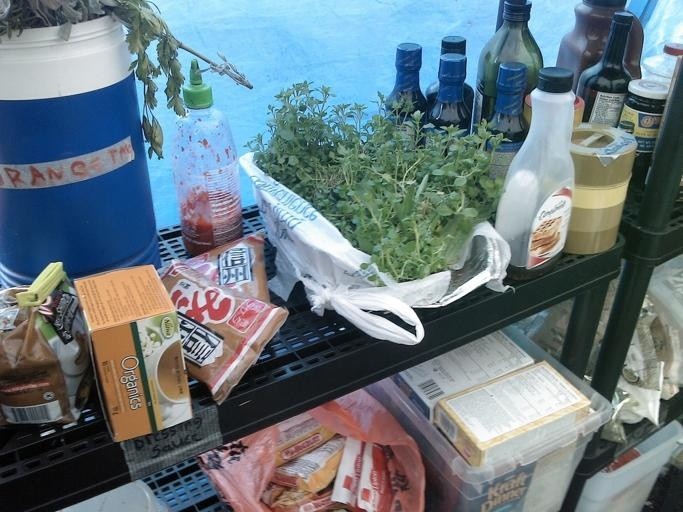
[559,50,683,512]
[0,200,626,512]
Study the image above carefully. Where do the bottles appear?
[383,42,429,149]
[616,78,672,165]
[423,52,472,156]
[575,10,634,128]
[169,58,244,255]
[641,41,683,86]
[424,35,476,112]
[472,1,545,131]
[474,60,530,229]
[494,66,577,269]
